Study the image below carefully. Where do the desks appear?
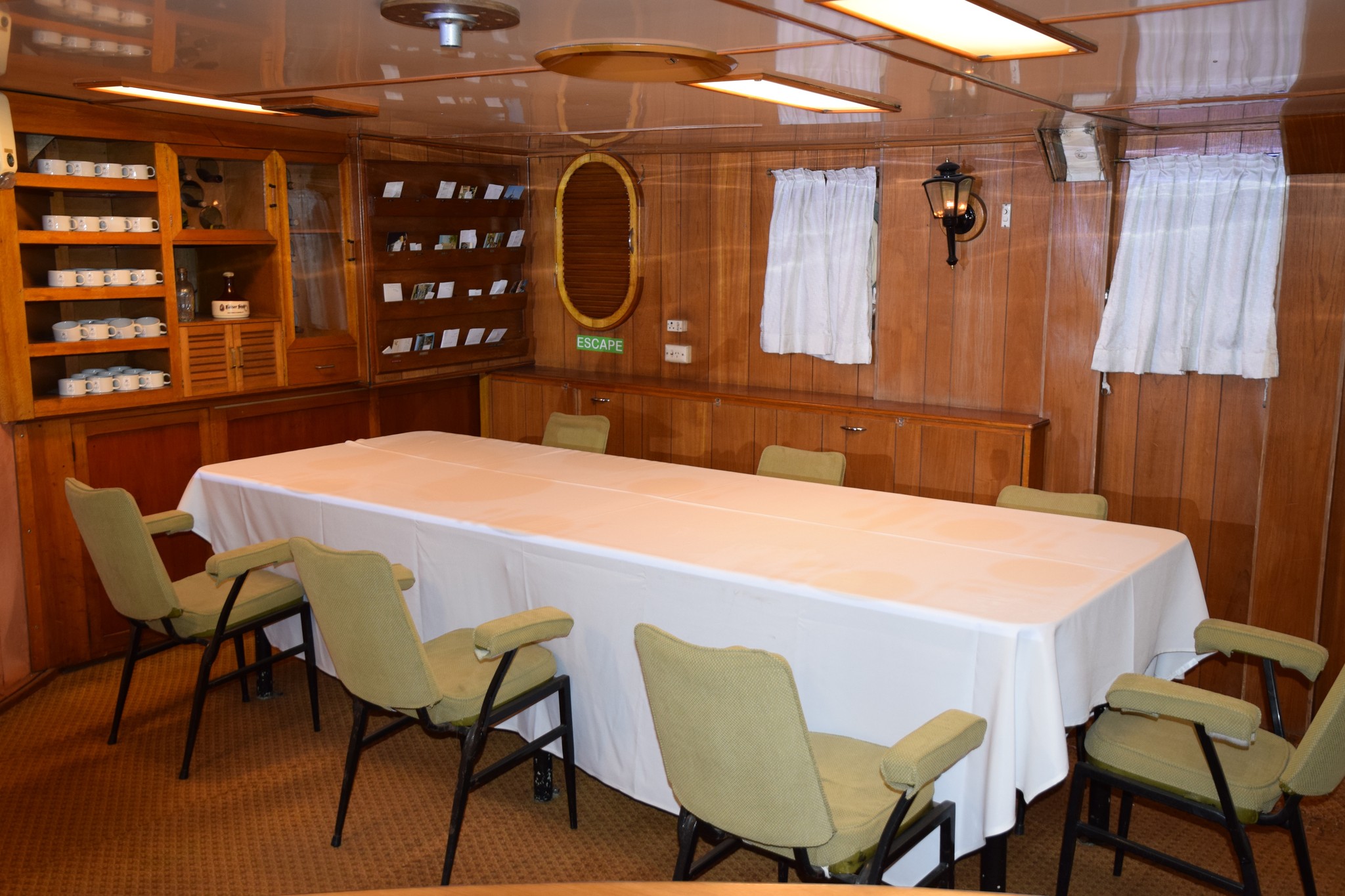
[178,431,1217,888]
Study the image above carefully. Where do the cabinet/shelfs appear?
[368,149,1049,506]
[0,88,366,712]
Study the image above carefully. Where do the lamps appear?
[922,158,987,270]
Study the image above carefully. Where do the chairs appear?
[69,412,1345,896]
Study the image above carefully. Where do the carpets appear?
[0,632,1345,896]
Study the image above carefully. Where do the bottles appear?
[211,272,250,319]
[176,267,194,322]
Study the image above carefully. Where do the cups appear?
[41,214,160,232]
[47,267,164,287]
[51,316,167,341]
[37,158,155,179]
[58,366,170,396]
